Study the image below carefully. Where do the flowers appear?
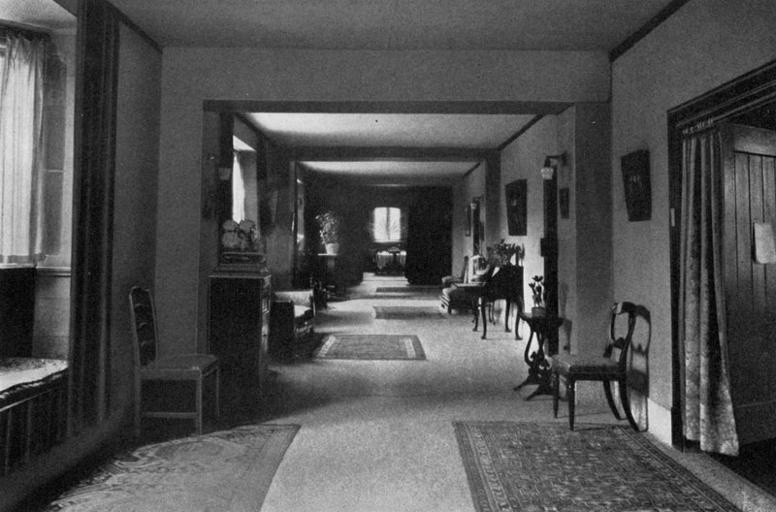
[528,275,558,308]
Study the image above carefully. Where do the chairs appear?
[127,284,223,437]
[271,290,316,356]
[549,301,640,432]
[440,256,523,342]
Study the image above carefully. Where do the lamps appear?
[540,151,570,181]
[469,196,484,210]
[207,151,231,183]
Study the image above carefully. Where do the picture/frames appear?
[461,206,471,236]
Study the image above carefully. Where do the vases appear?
[531,307,547,319]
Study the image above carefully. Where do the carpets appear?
[12,422,302,512]
[312,333,426,360]
[452,419,742,512]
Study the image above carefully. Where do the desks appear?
[514,312,563,404]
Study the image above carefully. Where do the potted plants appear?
[491,237,520,265]
[316,211,341,253]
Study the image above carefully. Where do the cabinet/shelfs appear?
[211,276,271,388]
